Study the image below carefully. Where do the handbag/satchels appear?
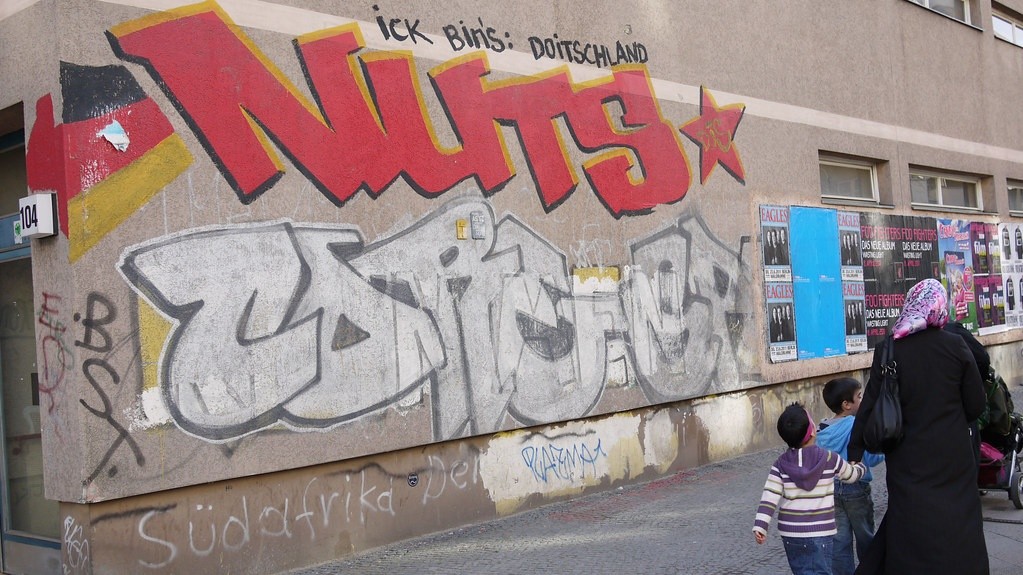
[863,334,902,453]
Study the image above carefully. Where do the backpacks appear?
[978,366,1014,436]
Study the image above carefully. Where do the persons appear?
[752,402,867,574]
[758,205,869,361]
[847,278,992,575]
[813,378,886,575]
[934,286,991,493]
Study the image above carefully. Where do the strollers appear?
[970,365,1023,512]
